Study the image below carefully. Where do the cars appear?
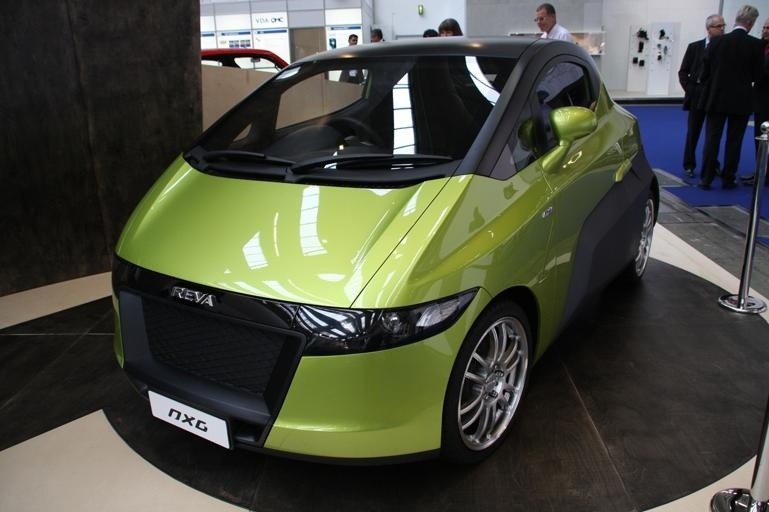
[109,35,664,476]
[198,46,293,77]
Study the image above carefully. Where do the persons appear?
[423,28,438,37]
[698,5,759,190]
[679,12,722,179]
[369,27,386,43]
[438,18,463,36]
[533,3,577,44]
[338,34,364,83]
[740,17,769,187]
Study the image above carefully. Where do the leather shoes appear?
[742,180,754,185]
[740,175,754,181]
[722,179,737,188]
[713,167,721,176]
[697,182,710,190]
[684,168,694,177]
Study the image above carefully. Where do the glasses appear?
[709,24,727,30]
